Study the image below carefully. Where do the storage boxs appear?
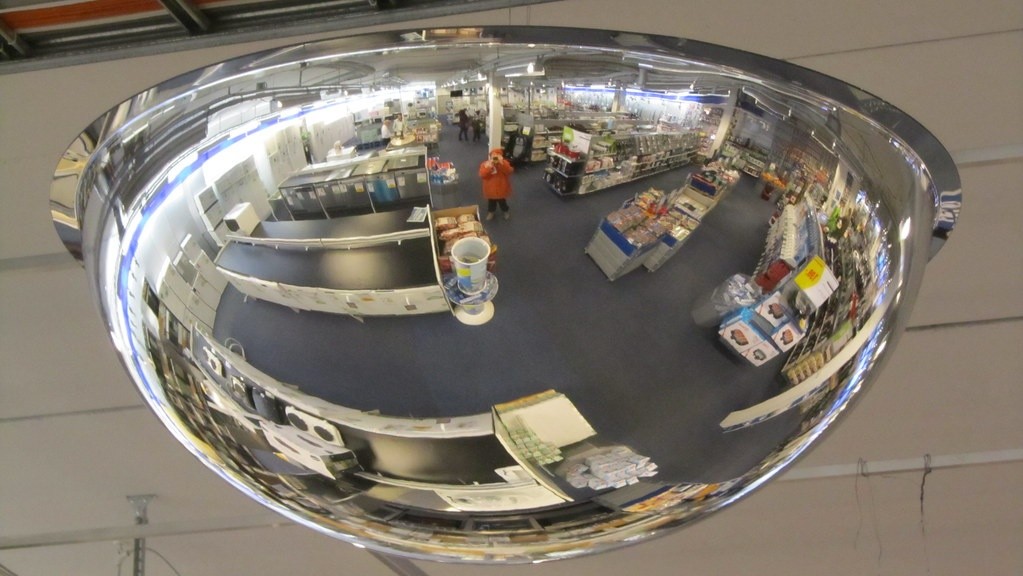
[430,204,497,273]
[545,154,583,194]
[154,121,326,336]
[717,255,840,367]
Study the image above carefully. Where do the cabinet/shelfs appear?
[780,245,857,384]
[543,130,699,199]
[516,112,656,169]
[743,159,763,178]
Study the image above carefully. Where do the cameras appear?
[493,156,499,160]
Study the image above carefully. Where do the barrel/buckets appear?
[450,236,491,293]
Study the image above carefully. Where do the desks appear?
[443,270,499,326]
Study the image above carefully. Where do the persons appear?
[382,120,391,146]
[393,112,405,137]
[459,110,469,141]
[480,148,512,220]
[472,117,481,142]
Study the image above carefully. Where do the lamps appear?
[742,86,792,121]
[811,124,837,154]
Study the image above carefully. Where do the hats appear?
[489,149,503,156]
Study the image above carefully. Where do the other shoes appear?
[502,211,510,220]
[486,212,495,221]
